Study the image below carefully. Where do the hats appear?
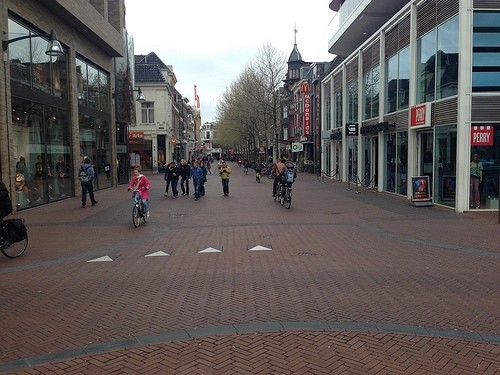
[285,161,294,168]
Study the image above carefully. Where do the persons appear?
[0,177,13,248]
[115,158,122,183]
[281,158,297,199]
[127,165,151,219]
[470,154,483,209]
[164,155,214,200]
[219,162,231,196]
[217,159,225,173]
[244,159,249,172]
[103,159,112,181]
[254,164,261,180]
[79,158,99,208]
[238,157,242,165]
[272,157,286,197]
[14,155,72,208]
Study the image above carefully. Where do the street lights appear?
[112,87,146,101]
[77,89,110,101]
[176,97,190,158]
[186,139,198,143]
[2,28,65,56]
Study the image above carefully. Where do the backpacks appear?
[80,164,92,182]
[285,169,294,183]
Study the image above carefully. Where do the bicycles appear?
[268,167,277,179]
[238,161,242,167]
[256,171,261,183]
[280,173,292,208]
[127,187,149,226]
[244,167,248,175]
[273,171,284,203]
[0,208,27,259]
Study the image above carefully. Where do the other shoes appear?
[173,191,179,199]
[272,195,276,198]
[193,193,196,195]
[181,192,185,195]
[82,205,85,208]
[92,201,98,204]
[202,191,205,195]
[146,211,150,217]
[185,193,189,196]
[164,192,168,196]
[222,194,229,197]
[195,194,200,199]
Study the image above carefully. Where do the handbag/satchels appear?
[6,220,27,242]
[129,185,138,200]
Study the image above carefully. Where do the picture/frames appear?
[442,175,456,201]
[411,176,430,201]
